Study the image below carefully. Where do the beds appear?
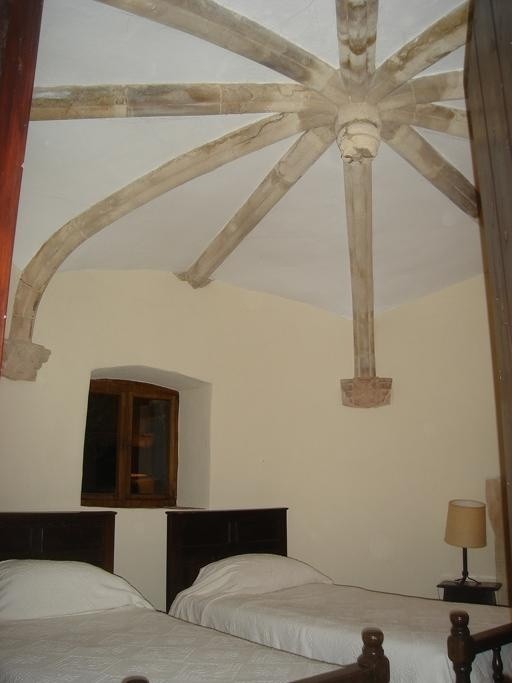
[1,511,390,682]
[164,507,512,681]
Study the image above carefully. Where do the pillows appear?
[195,552,332,597]
[0,559,143,621]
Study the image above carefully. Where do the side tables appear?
[436,581,501,603]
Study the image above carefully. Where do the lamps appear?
[446,501,486,582]
